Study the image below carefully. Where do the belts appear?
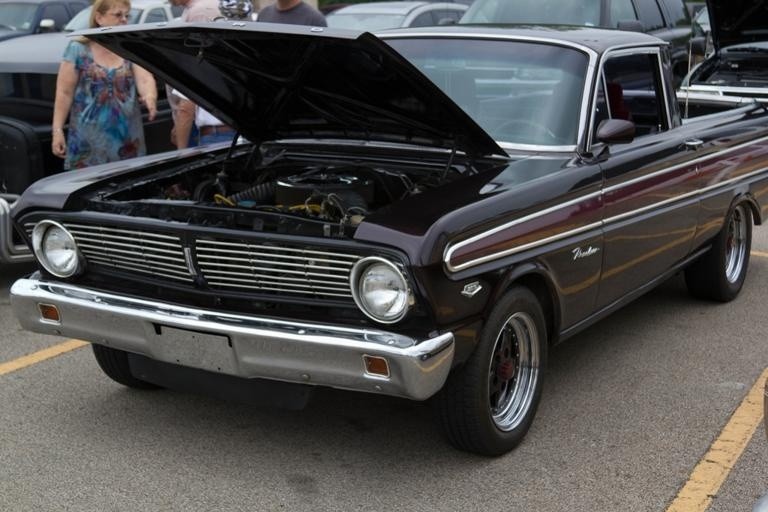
[196,122,235,138]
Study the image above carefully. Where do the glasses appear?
[107,10,132,21]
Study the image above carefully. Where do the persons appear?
[256,0,329,28]
[163,1,224,146]
[168,85,249,153]
[50,2,159,171]
